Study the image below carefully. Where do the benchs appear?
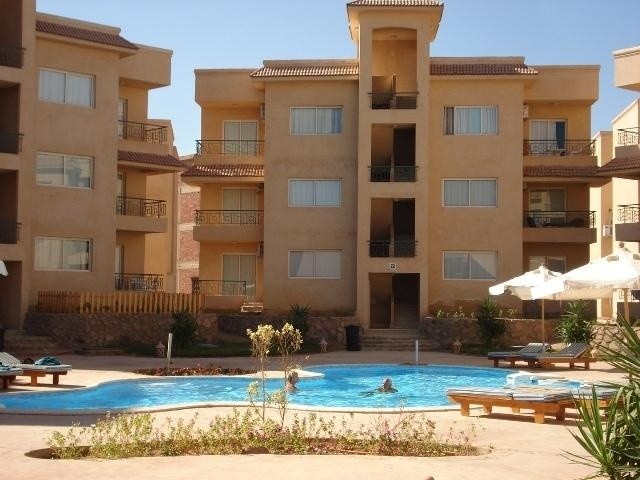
[446,385,630,425]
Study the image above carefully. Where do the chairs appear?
[489,338,591,369]
[0,352,72,389]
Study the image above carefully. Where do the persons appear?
[487,262,610,365]
[376,378,398,393]
[285,371,299,392]
[531,241,640,328]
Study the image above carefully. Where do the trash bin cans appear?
[344,325,361,350]
